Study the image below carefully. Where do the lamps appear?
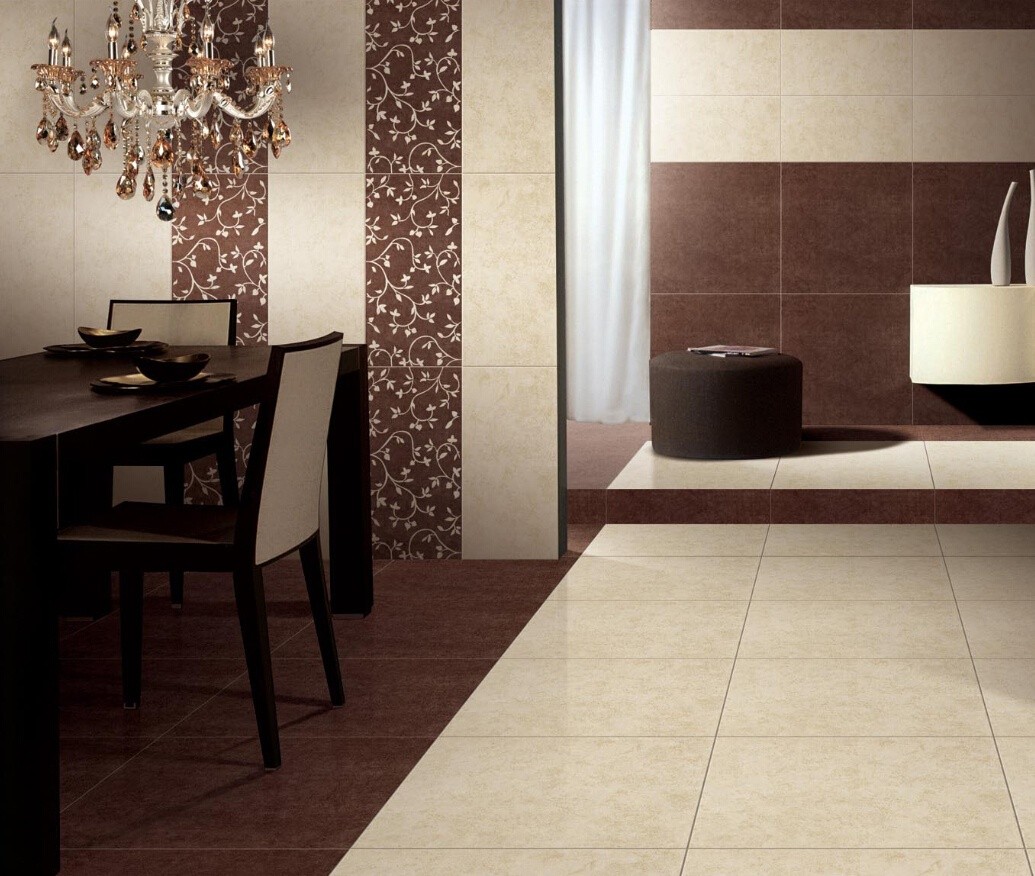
[22,0,297,224]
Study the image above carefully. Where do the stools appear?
[649,338,806,463]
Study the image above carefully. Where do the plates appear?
[89,373,236,392]
[46,341,168,353]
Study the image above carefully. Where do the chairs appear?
[100,295,238,610]
[43,324,355,774]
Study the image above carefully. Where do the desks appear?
[0,339,376,876]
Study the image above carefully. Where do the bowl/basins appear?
[133,353,211,382]
[77,327,142,347]
